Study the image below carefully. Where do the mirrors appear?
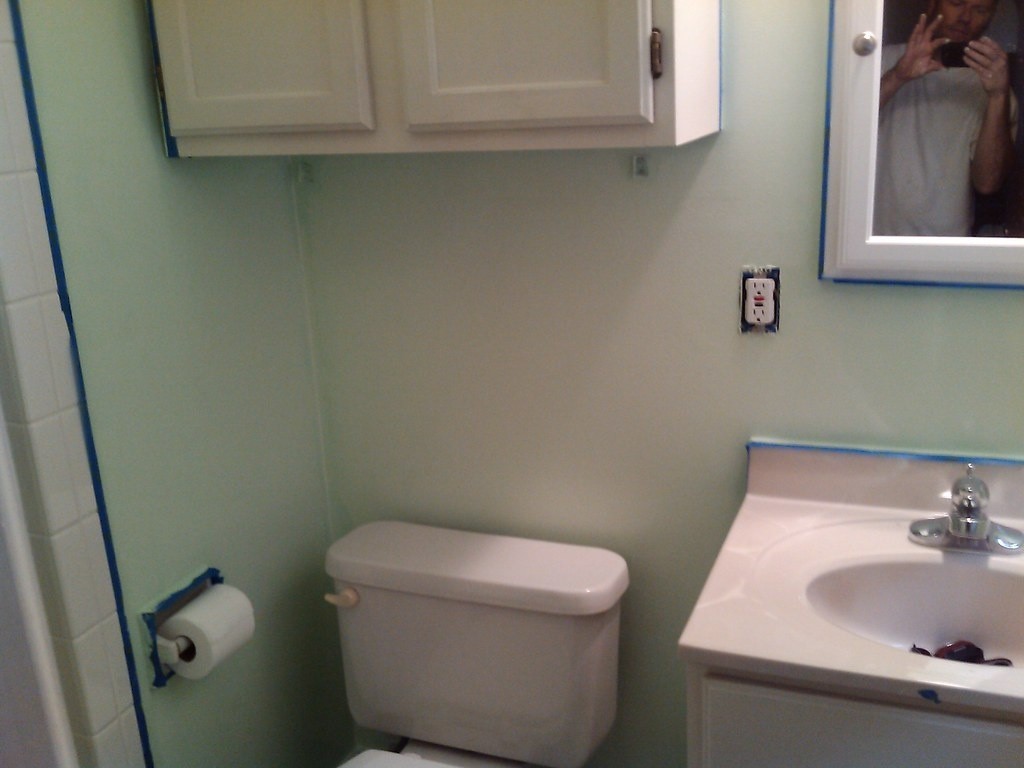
[819,0,1023,289]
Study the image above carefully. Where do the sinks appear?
[803,557,1024,669]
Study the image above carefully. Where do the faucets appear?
[908,460,1024,557]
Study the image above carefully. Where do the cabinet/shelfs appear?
[148,1,722,157]
[680,670,1024,768]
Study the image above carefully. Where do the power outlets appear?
[740,267,780,334]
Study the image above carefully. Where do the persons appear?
[872,1,1021,237]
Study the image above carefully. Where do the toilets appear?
[322,518,630,767]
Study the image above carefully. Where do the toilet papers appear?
[157,583,256,682]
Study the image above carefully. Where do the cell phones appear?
[940,41,973,68]
